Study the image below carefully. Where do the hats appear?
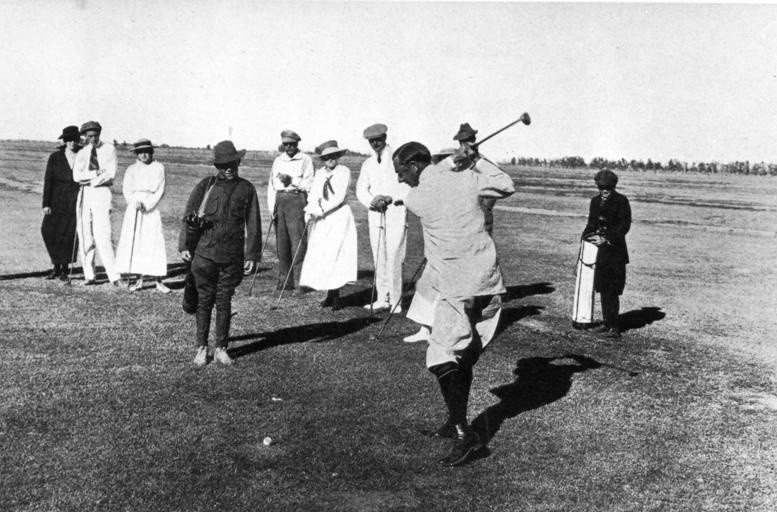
[452,122,478,141]
[281,129,302,144]
[58,125,80,140]
[212,139,247,165]
[362,123,388,139]
[311,139,347,159]
[80,121,102,136]
[130,138,159,152]
[594,170,619,191]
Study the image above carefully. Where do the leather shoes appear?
[48,268,171,294]
[276,283,342,311]
[603,323,620,339]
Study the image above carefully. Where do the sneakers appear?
[403,326,431,344]
[364,300,388,310]
[212,347,237,367]
[194,345,208,367]
[390,303,402,314]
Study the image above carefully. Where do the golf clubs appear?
[270,220,310,309]
[470,113,531,150]
[244,219,274,296]
[367,258,426,341]
[367,213,382,325]
[126,210,139,295]
[586,216,608,244]
[64,185,84,286]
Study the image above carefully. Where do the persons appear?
[390,122,514,468]
[41,126,86,283]
[178,142,261,368]
[581,169,632,338]
[113,137,172,295]
[72,121,125,289]
[268,127,358,312]
[356,124,408,316]
[403,123,498,343]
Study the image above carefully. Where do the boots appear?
[437,417,481,467]
[427,408,469,437]
